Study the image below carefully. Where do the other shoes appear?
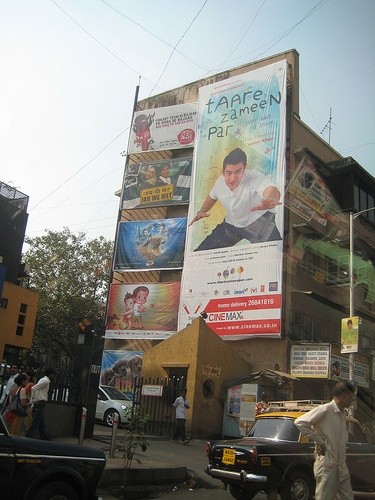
[183,440,189,445]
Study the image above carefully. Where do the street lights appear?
[348,208,375,381]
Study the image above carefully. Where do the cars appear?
[0,413,107,500]
[204,412,375,500]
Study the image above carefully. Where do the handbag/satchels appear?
[10,387,28,417]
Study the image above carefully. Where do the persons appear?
[137,163,182,199]
[167,389,190,445]
[331,361,340,377]
[343,319,358,349]
[136,224,169,246]
[293,382,356,500]
[188,148,282,252]
[0,363,57,441]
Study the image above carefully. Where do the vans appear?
[51,384,137,428]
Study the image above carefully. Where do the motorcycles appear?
[132,112,156,151]
